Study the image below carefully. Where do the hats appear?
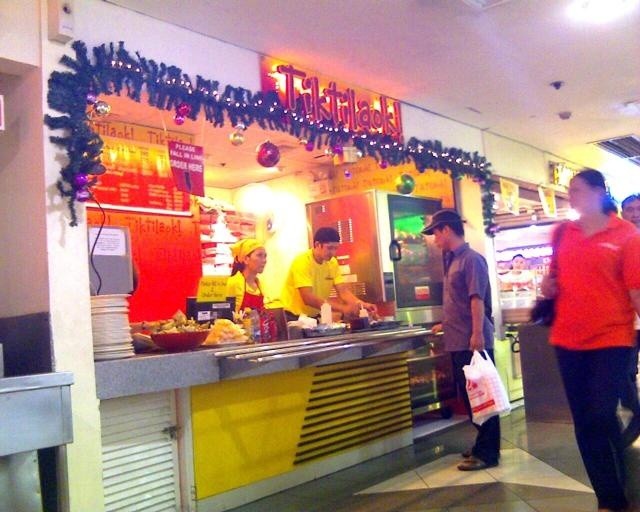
[421,209,460,235]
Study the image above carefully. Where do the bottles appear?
[320,297,332,327]
[359,302,369,321]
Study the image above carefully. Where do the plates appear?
[201,340,246,349]
[151,330,211,352]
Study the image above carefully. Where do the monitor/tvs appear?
[186,296,236,329]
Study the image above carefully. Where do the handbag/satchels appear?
[531,299,554,326]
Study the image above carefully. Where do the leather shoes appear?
[457,449,498,470]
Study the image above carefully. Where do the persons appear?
[224,239,267,314]
[541,169,640,512]
[282,227,378,321]
[502,253,531,283]
[621,194,640,448]
[421,210,501,471]
